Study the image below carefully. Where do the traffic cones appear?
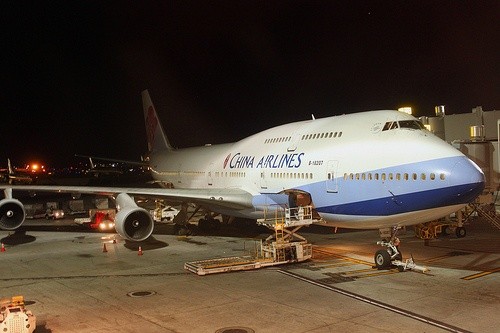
[113,234,116,243]
[138,245,143,256]
[0,242,6,252]
[103,243,108,252]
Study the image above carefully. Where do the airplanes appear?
[0,89,486,269]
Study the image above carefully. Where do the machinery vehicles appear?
[26,208,65,220]
[74,208,116,232]
